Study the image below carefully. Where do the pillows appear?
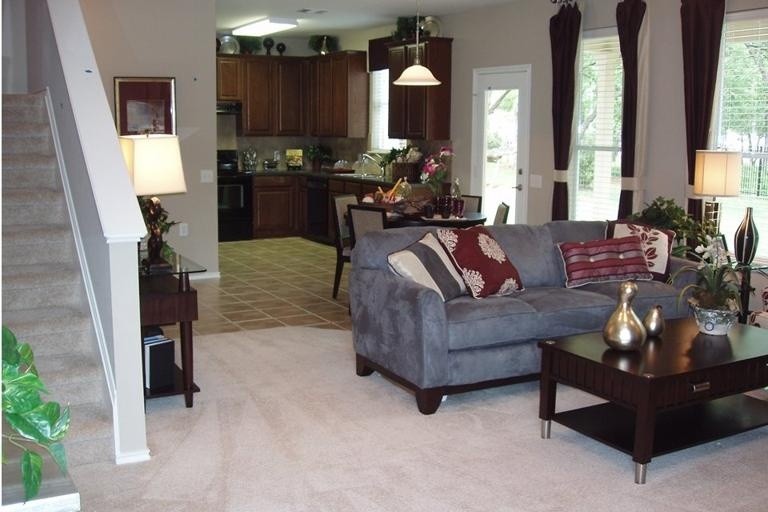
[387,220,677,303]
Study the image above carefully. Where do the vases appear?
[735,207,759,265]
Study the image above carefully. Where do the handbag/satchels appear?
[391,154,420,182]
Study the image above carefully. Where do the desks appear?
[138,240,207,407]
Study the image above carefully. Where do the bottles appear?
[264,160,268,170]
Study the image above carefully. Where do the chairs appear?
[330,177,510,317]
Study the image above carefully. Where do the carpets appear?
[81,325,768,512]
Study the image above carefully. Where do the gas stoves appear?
[218,171,251,177]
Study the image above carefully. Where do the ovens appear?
[218,174,253,242]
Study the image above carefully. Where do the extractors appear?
[216,104,240,114]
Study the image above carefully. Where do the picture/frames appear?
[113,76,177,135]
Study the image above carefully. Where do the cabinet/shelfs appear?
[253,175,297,239]
[297,174,308,238]
[388,37,454,142]
[216,50,369,137]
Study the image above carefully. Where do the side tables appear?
[665,257,768,335]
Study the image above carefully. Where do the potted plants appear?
[307,144,330,170]
[136,196,181,259]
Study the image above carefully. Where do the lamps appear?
[119,135,188,271]
[694,148,742,237]
[392,0,442,87]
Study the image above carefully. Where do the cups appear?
[441,208,452,219]
[425,205,435,219]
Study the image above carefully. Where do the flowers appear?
[419,142,456,195]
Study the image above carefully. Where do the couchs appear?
[348,221,712,415]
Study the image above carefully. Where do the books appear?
[142,334,176,392]
